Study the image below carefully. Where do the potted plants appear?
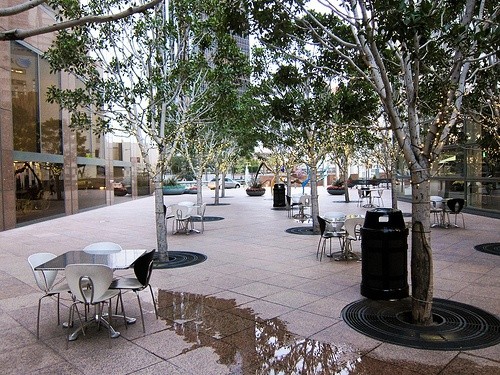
[246,178,265,196]
[164,178,186,194]
[327,178,345,195]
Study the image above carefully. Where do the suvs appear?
[448,181,489,205]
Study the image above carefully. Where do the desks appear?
[166,203,202,235]
[430,200,457,228]
[34,249,146,341]
[360,190,380,208]
[328,218,362,261]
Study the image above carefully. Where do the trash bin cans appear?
[273,184,286,207]
[359,207,410,300]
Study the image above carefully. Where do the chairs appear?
[354,186,387,209]
[164,201,207,236]
[27,242,157,350]
[285,194,312,221]
[431,196,467,230]
[315,215,365,263]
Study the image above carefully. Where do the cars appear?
[208,176,240,190]
